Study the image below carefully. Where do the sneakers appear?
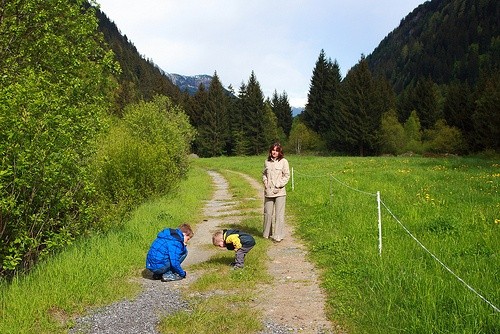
[152,272,162,280]
[161,270,183,282]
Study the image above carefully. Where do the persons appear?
[262,143,290,242]
[213,229,256,269]
[146,224,194,282]
